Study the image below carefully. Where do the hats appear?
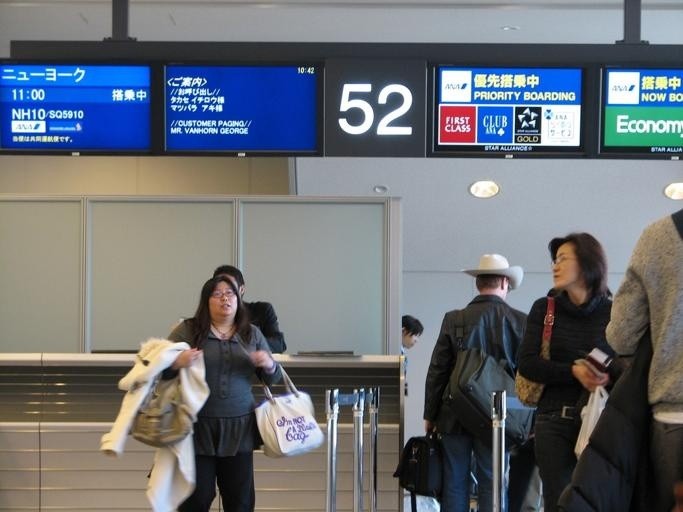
[459,253,525,291]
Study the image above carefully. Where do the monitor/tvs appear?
[426,58,683,160]
[0,58,324,157]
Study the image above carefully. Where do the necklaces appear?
[212,323,234,339]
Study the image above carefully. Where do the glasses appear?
[210,289,235,298]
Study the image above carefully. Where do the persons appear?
[401,315,423,382]
[604,207,683,512]
[162,274,282,512]
[213,265,287,353]
[424,255,533,512]
[517,233,622,512]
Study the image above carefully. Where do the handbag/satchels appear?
[131,372,192,447]
[254,365,325,457]
[396,429,442,500]
[443,348,535,446]
[516,343,550,408]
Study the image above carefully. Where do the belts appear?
[539,402,580,419]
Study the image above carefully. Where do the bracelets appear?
[266,362,275,372]
